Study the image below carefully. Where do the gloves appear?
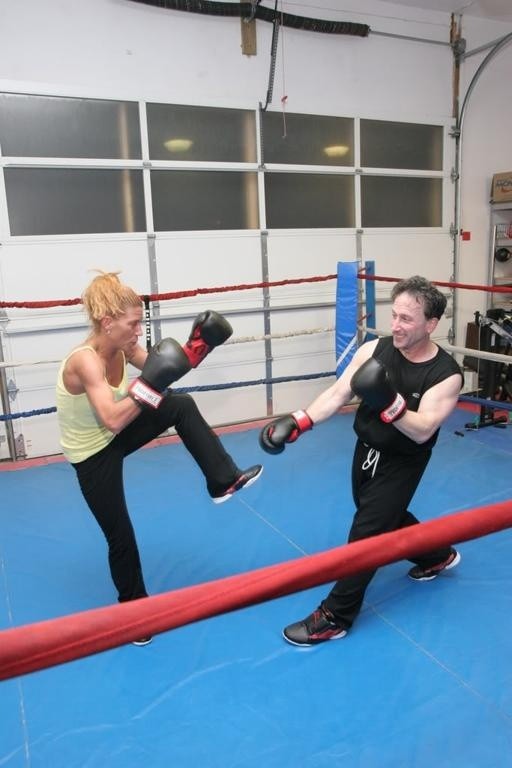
[259,409,313,455]
[126,310,233,411]
[350,358,407,423]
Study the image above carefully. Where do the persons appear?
[57,271,263,647]
[259,275,464,647]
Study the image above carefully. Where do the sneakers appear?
[283,600,348,646]
[408,546,461,580]
[133,637,153,645]
[211,464,264,505]
